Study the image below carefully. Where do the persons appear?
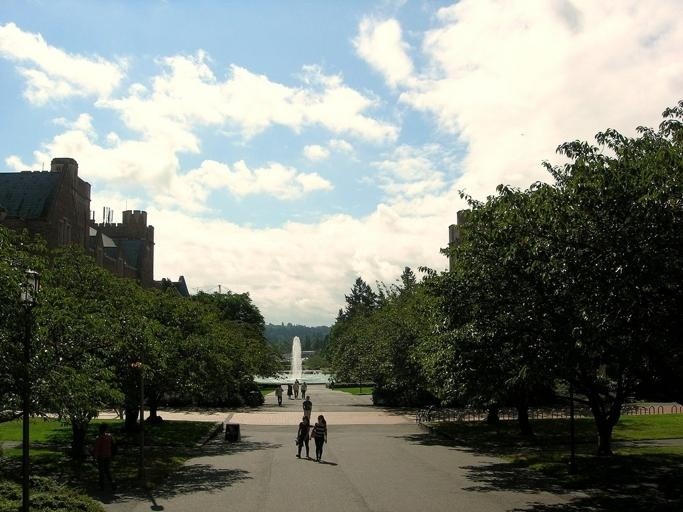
[302,395,312,420]
[287,379,307,399]
[331,381,334,390]
[310,415,327,462]
[92,422,116,492]
[275,385,284,407]
[295,415,311,460]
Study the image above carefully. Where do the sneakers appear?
[295,454,321,463]
